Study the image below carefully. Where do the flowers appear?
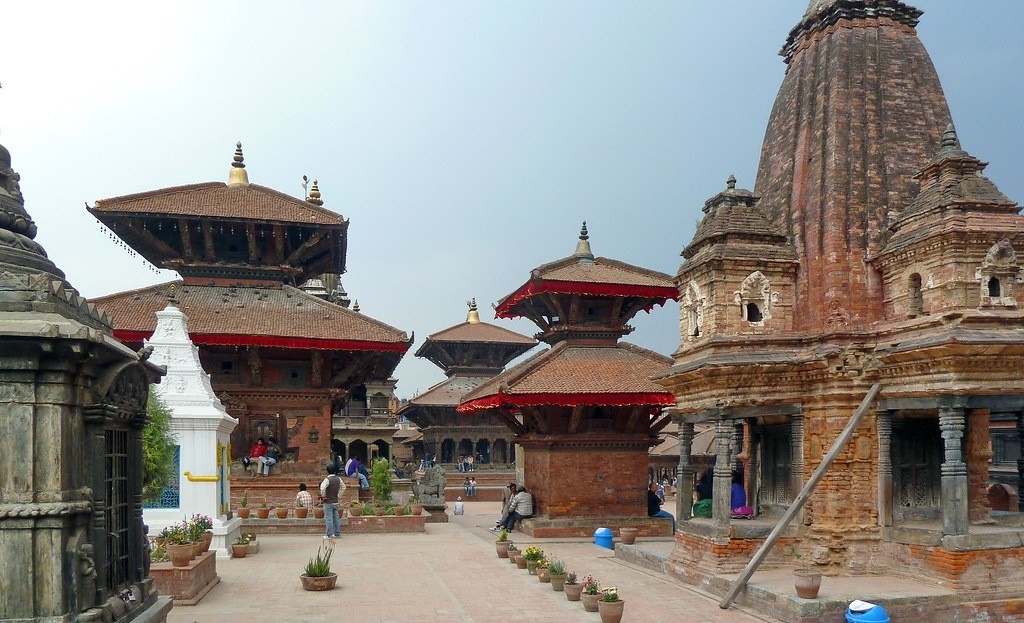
[167,511,213,544]
[303,532,335,577]
[583,574,601,594]
[602,586,619,603]
[563,570,577,586]
[523,545,544,561]
[508,542,517,551]
[235,533,252,545]
[348,499,362,507]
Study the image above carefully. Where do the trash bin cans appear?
[594,527,613,550]
[845,599,891,623]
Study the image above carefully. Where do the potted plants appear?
[494,528,513,558]
[237,494,424,519]
[548,559,568,591]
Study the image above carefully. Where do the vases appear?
[620,527,638,544]
[300,571,338,591]
[349,506,364,516]
[232,543,248,558]
[793,568,823,599]
[507,549,625,623]
[166,531,213,566]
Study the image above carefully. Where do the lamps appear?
[308,425,319,443]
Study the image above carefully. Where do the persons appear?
[463,477,477,497]
[648,466,746,534]
[143,526,153,579]
[418,453,437,472]
[79,544,98,612]
[457,449,483,472]
[296,483,313,512]
[243,437,283,477]
[454,496,464,515]
[331,441,372,491]
[320,464,346,539]
[490,482,534,533]
[392,456,403,479]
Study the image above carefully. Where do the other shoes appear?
[322,535,332,540]
[332,534,340,539]
[490,519,512,533]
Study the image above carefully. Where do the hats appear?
[507,483,516,487]
